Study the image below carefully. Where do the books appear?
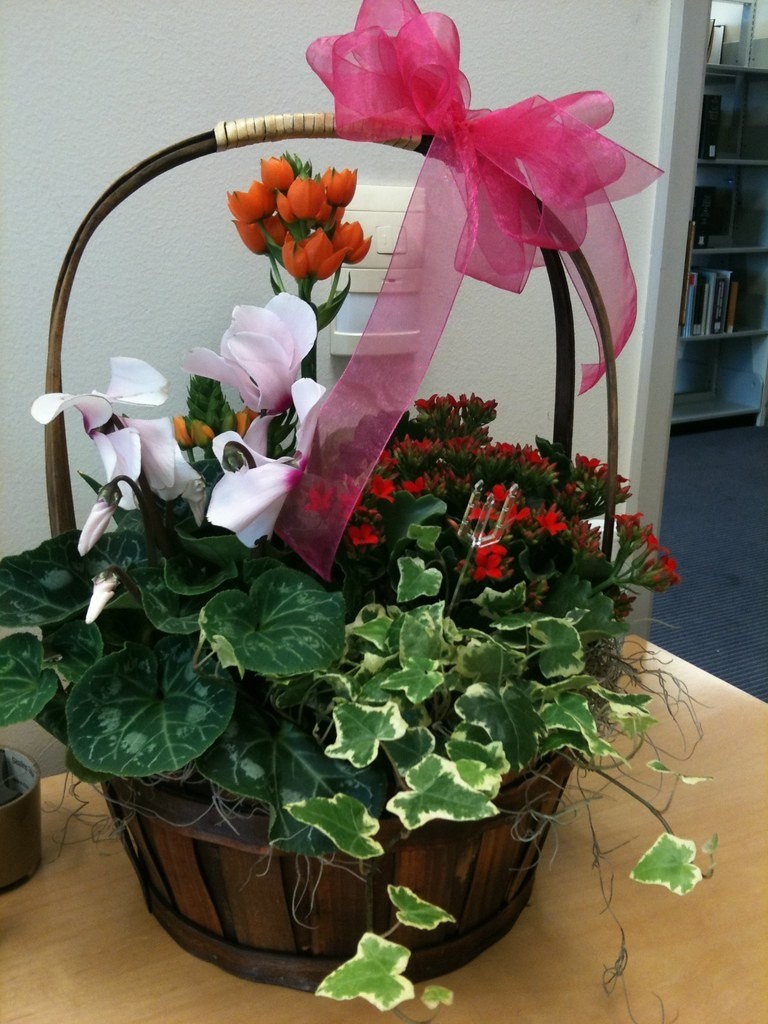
[692,185,715,249]
[681,266,738,338]
[698,94,722,160]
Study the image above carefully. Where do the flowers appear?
[0,152,719,1024]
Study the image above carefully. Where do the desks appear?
[0,634,768,1024]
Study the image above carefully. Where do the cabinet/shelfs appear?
[670,0,768,427]
